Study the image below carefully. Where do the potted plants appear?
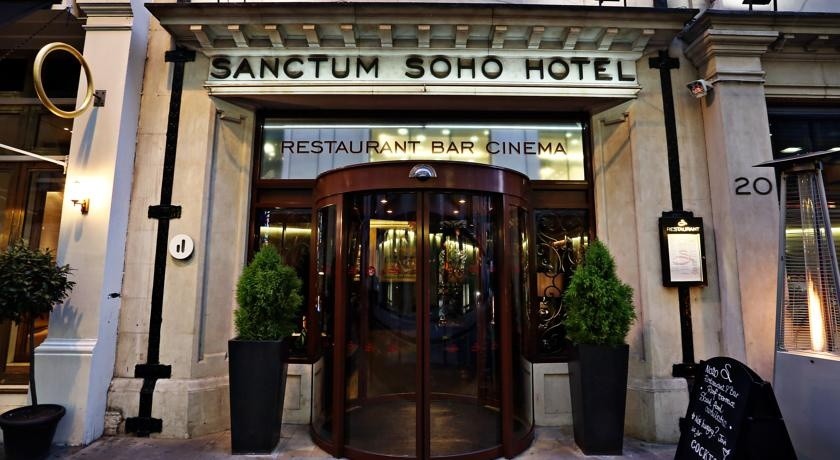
[562,238,639,453]
[227,239,305,454]
[0,239,77,460]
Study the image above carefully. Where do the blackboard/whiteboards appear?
[674,356,797,460]
